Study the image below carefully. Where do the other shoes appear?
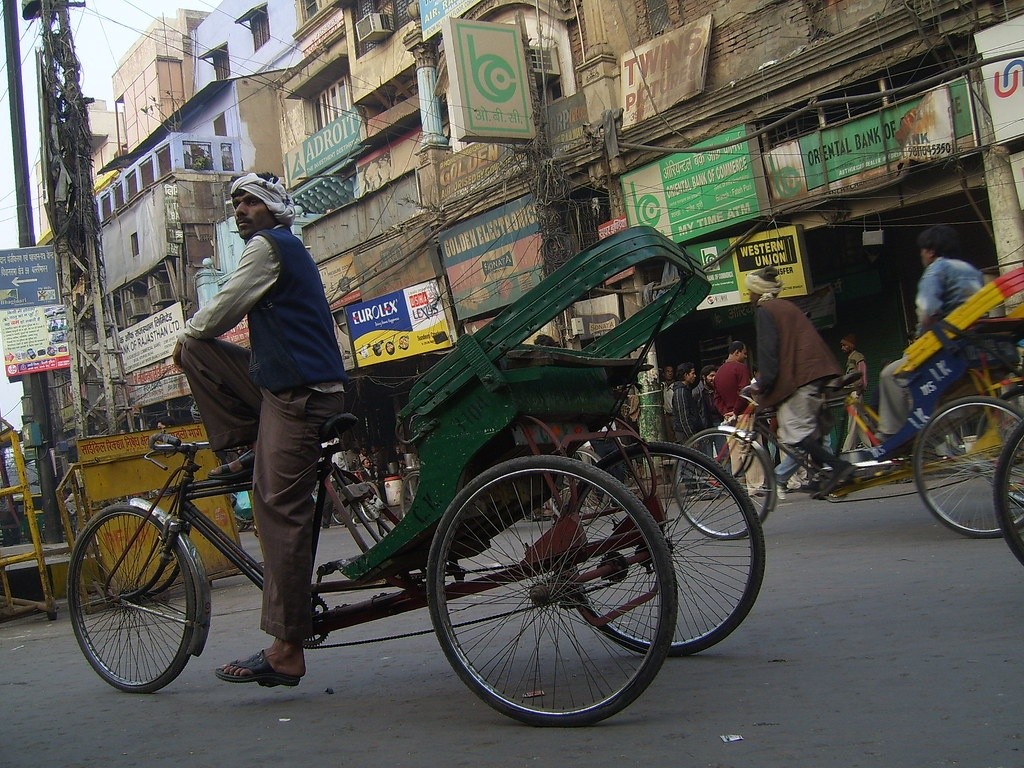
[777,484,786,500]
[874,431,892,442]
[788,485,808,493]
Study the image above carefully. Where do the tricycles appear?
[671,265,1024,537]
[66,225,767,728]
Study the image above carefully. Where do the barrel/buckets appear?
[384,476,403,506]
[387,461,398,474]
[404,454,417,466]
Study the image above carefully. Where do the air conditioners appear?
[148,282,173,305]
[124,297,146,319]
[355,12,393,43]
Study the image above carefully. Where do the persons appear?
[534,334,555,346]
[660,365,676,442]
[590,421,625,507]
[840,335,871,452]
[173,172,349,686]
[872,225,984,447]
[749,361,776,462]
[746,267,856,498]
[64,488,77,526]
[350,446,387,501]
[672,362,700,486]
[693,365,731,476]
[715,340,766,497]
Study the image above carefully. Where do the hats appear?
[840,334,857,349]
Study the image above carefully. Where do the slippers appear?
[208,448,258,479]
[215,649,300,686]
